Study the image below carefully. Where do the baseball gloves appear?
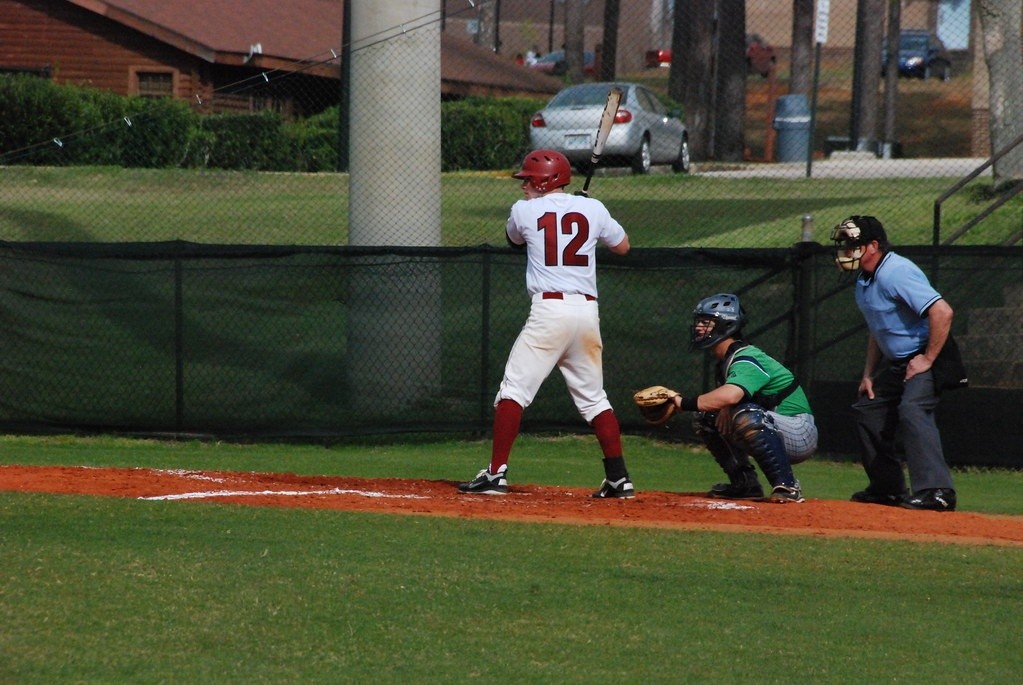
[633,384,682,425]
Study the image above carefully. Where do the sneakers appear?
[458,469,508,496]
[708,483,765,501]
[850,486,911,506]
[902,486,956,512]
[592,474,636,500]
[771,488,805,504]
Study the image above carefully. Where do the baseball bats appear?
[582,87,624,193]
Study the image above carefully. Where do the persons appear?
[634,293,819,503]
[830,215,956,512]
[456,151,631,500]
[514,45,541,68]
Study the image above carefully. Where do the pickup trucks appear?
[643,48,671,68]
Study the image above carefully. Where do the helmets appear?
[689,293,746,350]
[512,150,571,193]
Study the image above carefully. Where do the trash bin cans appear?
[772,95,812,164]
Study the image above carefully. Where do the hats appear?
[831,216,887,246]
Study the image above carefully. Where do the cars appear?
[709,32,778,79]
[529,81,690,176]
[528,48,597,78]
[880,28,952,81]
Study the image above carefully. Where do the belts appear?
[543,291,596,300]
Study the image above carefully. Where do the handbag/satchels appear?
[931,336,968,395]
